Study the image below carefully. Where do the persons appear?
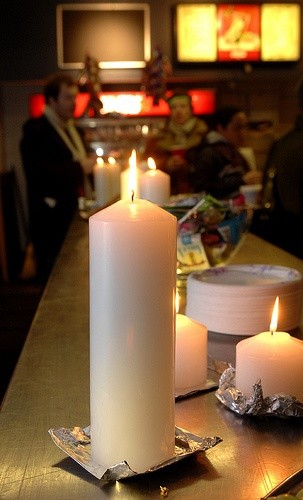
[141,94,207,195]
[185,106,251,202]
[20,75,99,294]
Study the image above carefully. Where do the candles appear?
[86,149,176,468]
[234,294,303,401]
[142,158,172,205]
[174,288,208,390]
[106,155,122,199]
[121,149,142,198]
[95,155,108,202]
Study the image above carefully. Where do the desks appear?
[0,209,303,500]
